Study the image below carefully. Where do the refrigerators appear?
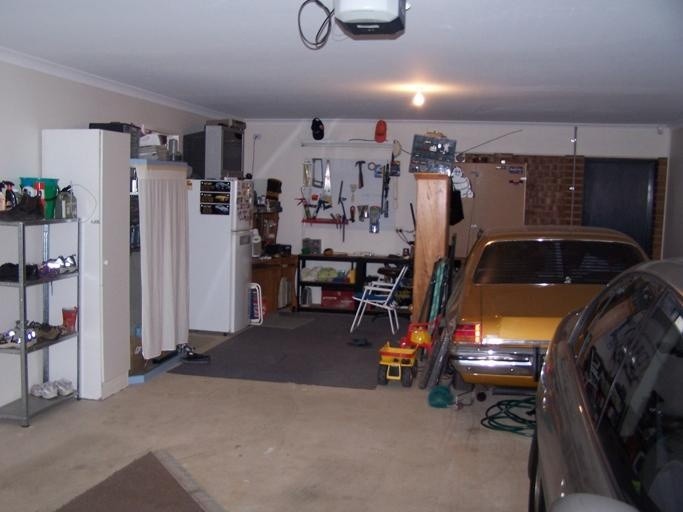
[186,178,254,335]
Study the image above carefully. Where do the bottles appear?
[34,181,45,220]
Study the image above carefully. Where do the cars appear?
[441,224,650,403]
[527,259,682,512]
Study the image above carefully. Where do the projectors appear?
[333,0,407,36]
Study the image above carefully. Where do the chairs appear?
[349,263,408,334]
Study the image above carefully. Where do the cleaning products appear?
[0,182,6,211]
[1,180,16,211]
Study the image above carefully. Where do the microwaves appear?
[250,235,262,258]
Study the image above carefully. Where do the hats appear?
[375,120,386,142]
[311,118,324,140]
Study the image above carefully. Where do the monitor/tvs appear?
[183,125,245,181]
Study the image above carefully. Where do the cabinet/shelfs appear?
[296,254,413,318]
[0,216,80,427]
[41,128,131,401]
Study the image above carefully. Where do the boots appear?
[0,193,43,221]
[60,308,76,335]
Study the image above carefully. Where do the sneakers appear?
[0,320,61,348]
[0,256,76,280]
[31,382,57,399]
[180,353,210,364]
[177,344,195,354]
[55,379,75,396]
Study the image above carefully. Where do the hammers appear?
[355,160,365,189]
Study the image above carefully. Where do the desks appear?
[251,255,298,320]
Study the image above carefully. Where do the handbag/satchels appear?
[450,178,464,226]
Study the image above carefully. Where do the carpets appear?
[53,448,223,511]
[164,310,410,391]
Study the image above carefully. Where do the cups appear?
[61,306,76,332]
[403,248,410,257]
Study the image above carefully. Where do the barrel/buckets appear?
[19,173,61,220]
[54,190,77,219]
